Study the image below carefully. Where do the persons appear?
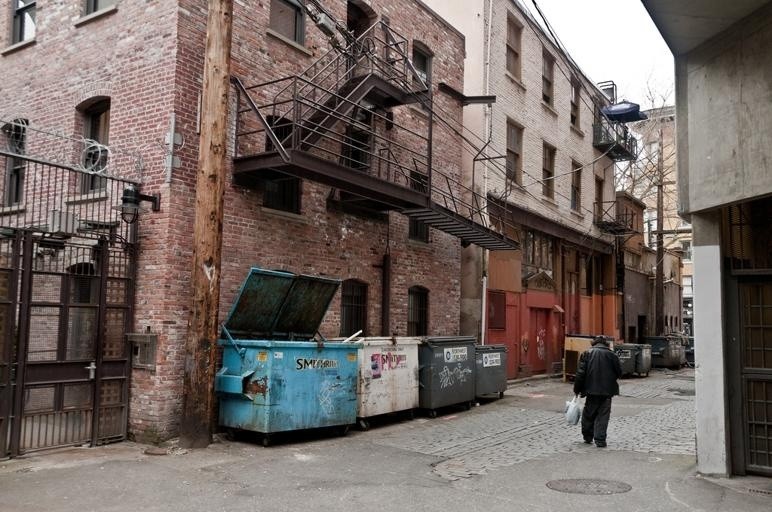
[573,335,622,447]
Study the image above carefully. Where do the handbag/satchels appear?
[566,393,580,425]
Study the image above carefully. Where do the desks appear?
[562,334,616,384]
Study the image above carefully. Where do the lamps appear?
[648,267,656,279]
[111,183,161,225]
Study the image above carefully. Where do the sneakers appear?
[583,440,605,447]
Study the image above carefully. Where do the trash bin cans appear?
[215,267,359,446]
[474,345,509,404]
[614,344,652,379]
[645,336,695,369]
[417,336,476,417]
[325,335,420,425]
